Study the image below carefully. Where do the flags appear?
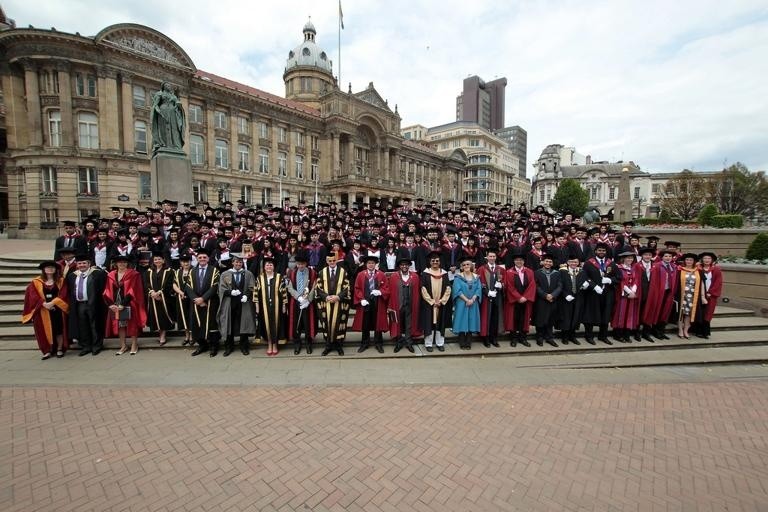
[340,0,345,31]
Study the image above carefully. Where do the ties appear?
[331,269,335,290]
[199,267,205,289]
[491,267,494,282]
[234,272,240,288]
[369,271,375,291]
[78,273,84,301]
[646,263,650,282]
[519,269,523,283]
[601,260,604,272]
[572,268,576,294]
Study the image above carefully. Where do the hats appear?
[60,221,79,235]
[37,260,61,269]
[679,253,698,270]
[403,198,411,201]
[417,199,424,201]
[658,251,677,263]
[282,197,293,200]
[664,241,681,252]
[530,206,660,259]
[75,253,93,261]
[96,201,529,266]
[699,253,717,268]
[81,219,98,228]
[57,248,78,252]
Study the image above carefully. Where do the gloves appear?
[298,296,306,303]
[583,281,589,289]
[361,300,369,306]
[595,285,603,294]
[602,277,612,284]
[623,284,637,294]
[230,290,241,295]
[300,301,310,310]
[488,290,497,297]
[370,290,382,296]
[565,295,575,301]
[241,295,248,303]
[495,282,502,288]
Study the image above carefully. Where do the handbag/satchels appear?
[111,306,131,320]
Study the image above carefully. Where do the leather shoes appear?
[394,341,531,353]
[183,339,194,345]
[696,334,711,338]
[115,346,138,354]
[191,345,384,356]
[159,342,166,346]
[42,350,100,360]
[679,336,691,339]
[538,334,670,347]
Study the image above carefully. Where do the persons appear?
[22,198,725,359]
[150,80,187,150]
